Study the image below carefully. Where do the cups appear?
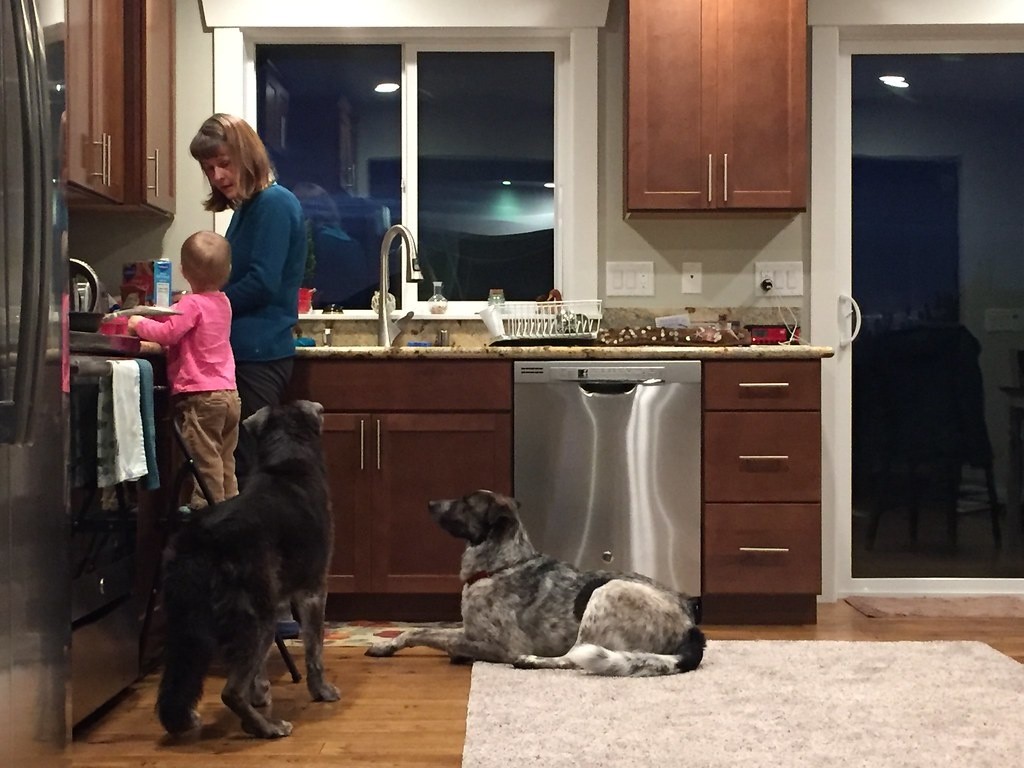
[298,289,316,314]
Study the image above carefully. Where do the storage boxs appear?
[122,258,172,307]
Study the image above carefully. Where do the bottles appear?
[427,282,448,314]
[489,289,505,306]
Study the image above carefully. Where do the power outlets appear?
[755,261,804,297]
[984,308,1023,333]
[606,261,655,296]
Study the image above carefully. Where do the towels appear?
[121,358,161,506]
[97,358,148,489]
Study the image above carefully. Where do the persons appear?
[126,230,241,513]
[189,112,310,638]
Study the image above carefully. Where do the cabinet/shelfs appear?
[64,0,125,205]
[622,0,808,212]
[299,359,514,617]
[702,358,822,624]
[68,0,177,219]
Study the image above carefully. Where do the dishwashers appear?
[514,359,701,598]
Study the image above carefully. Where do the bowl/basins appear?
[69,311,105,333]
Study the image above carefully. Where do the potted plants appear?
[299,220,315,313]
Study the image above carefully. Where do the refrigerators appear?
[0,0,70,768]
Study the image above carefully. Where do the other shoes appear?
[275,600,300,637]
[179,505,193,515]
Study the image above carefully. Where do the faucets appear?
[378,224,424,347]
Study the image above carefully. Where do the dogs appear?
[365,489,706,677]
[156,400,342,738]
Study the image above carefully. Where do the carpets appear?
[461,640,1024,768]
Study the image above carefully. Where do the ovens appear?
[69,354,143,728]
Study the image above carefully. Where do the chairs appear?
[138,401,303,683]
[852,324,1003,552]
